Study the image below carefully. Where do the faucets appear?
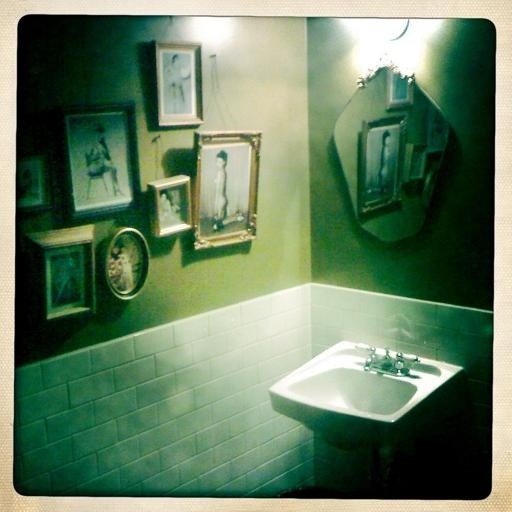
[354,344,423,378]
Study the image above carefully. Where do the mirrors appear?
[331,63,452,247]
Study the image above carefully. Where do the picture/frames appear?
[357,113,407,215]
[17,152,54,214]
[148,175,194,238]
[97,226,152,303]
[27,223,98,320]
[422,168,440,205]
[426,100,447,154]
[385,69,413,111]
[194,131,262,251]
[401,143,428,182]
[155,42,205,127]
[62,102,143,225]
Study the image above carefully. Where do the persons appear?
[158,188,186,231]
[164,53,191,113]
[367,129,392,194]
[84,121,124,197]
[210,150,245,231]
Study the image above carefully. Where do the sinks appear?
[292,367,420,416]
